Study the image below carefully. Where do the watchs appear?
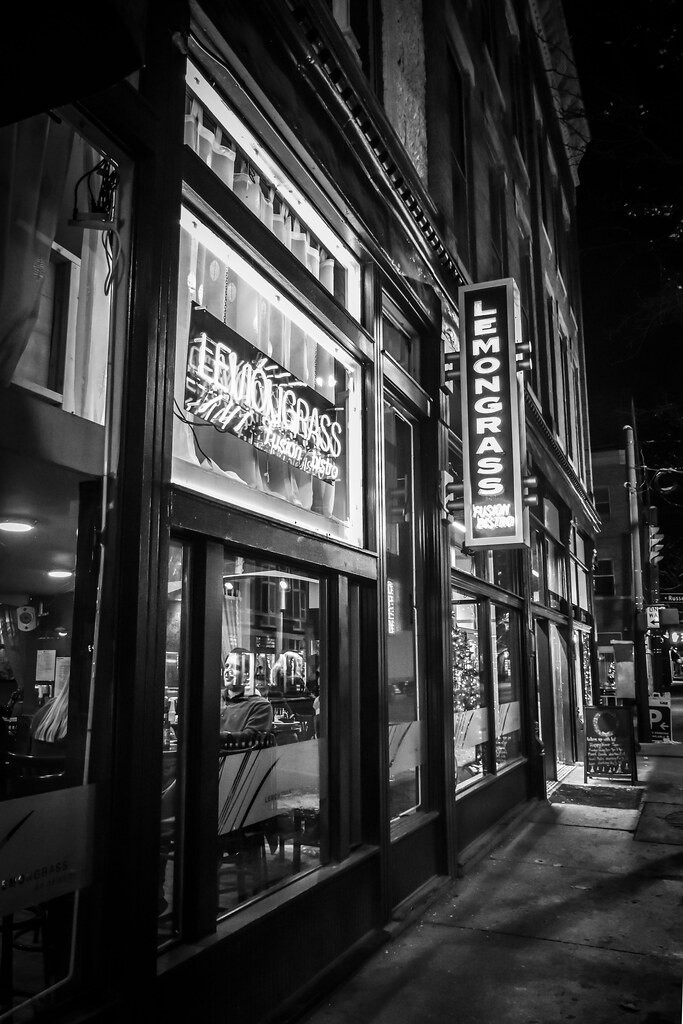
[226,731,233,743]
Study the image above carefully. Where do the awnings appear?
[671,649,681,661]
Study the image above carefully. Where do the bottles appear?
[164,695,171,750]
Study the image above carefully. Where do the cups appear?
[273,707,285,722]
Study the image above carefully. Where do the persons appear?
[269,650,321,742]
[28,672,70,777]
[256,666,265,686]
[158,647,274,916]
[0,662,19,718]
